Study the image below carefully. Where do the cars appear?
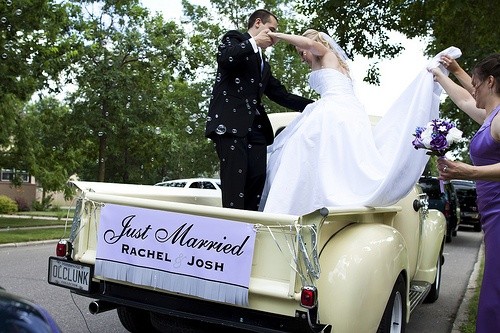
[155,177,221,189]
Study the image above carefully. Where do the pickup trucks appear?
[48,112,448,333]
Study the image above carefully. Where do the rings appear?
[443,61,446,64]
[443,166,447,172]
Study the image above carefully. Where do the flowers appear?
[261,46,275,62]
[411,119,466,203]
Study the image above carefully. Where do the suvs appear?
[418,176,461,243]
[449,178,482,232]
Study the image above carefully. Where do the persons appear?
[429,54,500,333]
[205,9,315,211]
[259,29,462,216]
[439,55,476,96]
